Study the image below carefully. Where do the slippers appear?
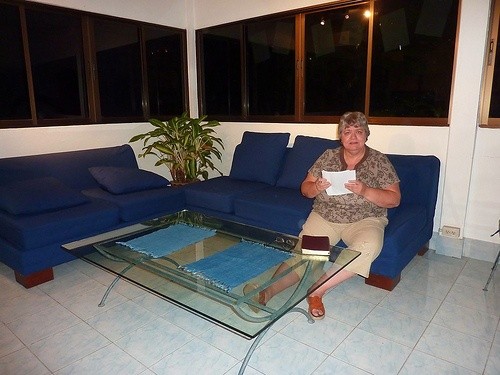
[244,282,266,312]
[306,293,326,320]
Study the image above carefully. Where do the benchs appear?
[182,143,443,292]
[0,144,180,290]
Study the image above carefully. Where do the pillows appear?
[87,165,171,196]
[277,135,342,191]
[228,131,291,186]
[0,175,91,218]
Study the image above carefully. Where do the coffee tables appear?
[58,208,362,375]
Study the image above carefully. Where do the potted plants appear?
[128,111,225,189]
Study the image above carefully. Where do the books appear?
[301,235,331,256]
[302,254,330,262]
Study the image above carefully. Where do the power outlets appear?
[441,225,461,239]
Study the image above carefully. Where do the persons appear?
[244,112,401,320]
